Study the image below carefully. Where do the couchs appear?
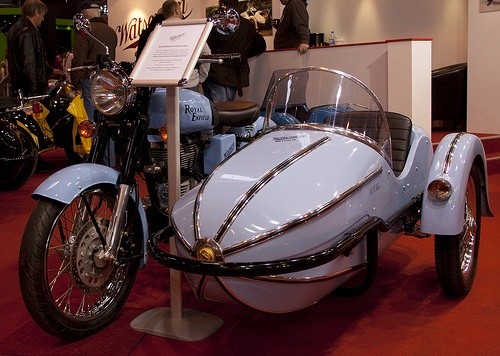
[431,62,467,130]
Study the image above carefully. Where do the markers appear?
[331,31,333,36]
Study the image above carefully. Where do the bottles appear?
[328,31,335,46]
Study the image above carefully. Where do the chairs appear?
[324,110,413,177]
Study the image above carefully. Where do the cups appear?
[273,19,280,28]
[309,33,324,46]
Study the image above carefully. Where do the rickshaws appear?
[17,5,491,343]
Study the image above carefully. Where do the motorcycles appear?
[0,53,139,189]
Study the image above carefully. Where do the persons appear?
[201,0,267,103]
[46,45,75,83]
[68,0,120,170]
[6,0,66,98]
[133,0,211,98]
[270,0,310,54]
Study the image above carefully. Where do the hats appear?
[80,1,100,9]
